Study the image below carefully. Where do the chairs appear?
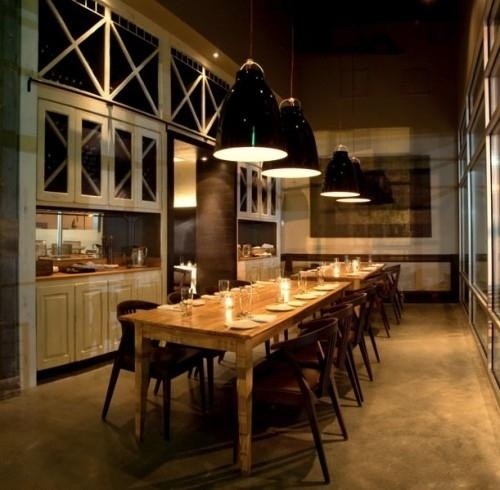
[101,299,215,442]
[217,279,270,365]
[226,318,348,482]
[291,263,404,408]
[152,291,217,396]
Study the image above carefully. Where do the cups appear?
[157,255,385,329]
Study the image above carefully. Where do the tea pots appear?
[121,245,150,268]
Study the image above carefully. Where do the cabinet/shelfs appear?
[237,162,261,221]
[261,164,278,223]
[37,275,108,381]
[36,83,109,210]
[108,104,162,213]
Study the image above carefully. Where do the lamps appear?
[319,52,371,203]
[212,0,289,162]
[261,34,322,179]
